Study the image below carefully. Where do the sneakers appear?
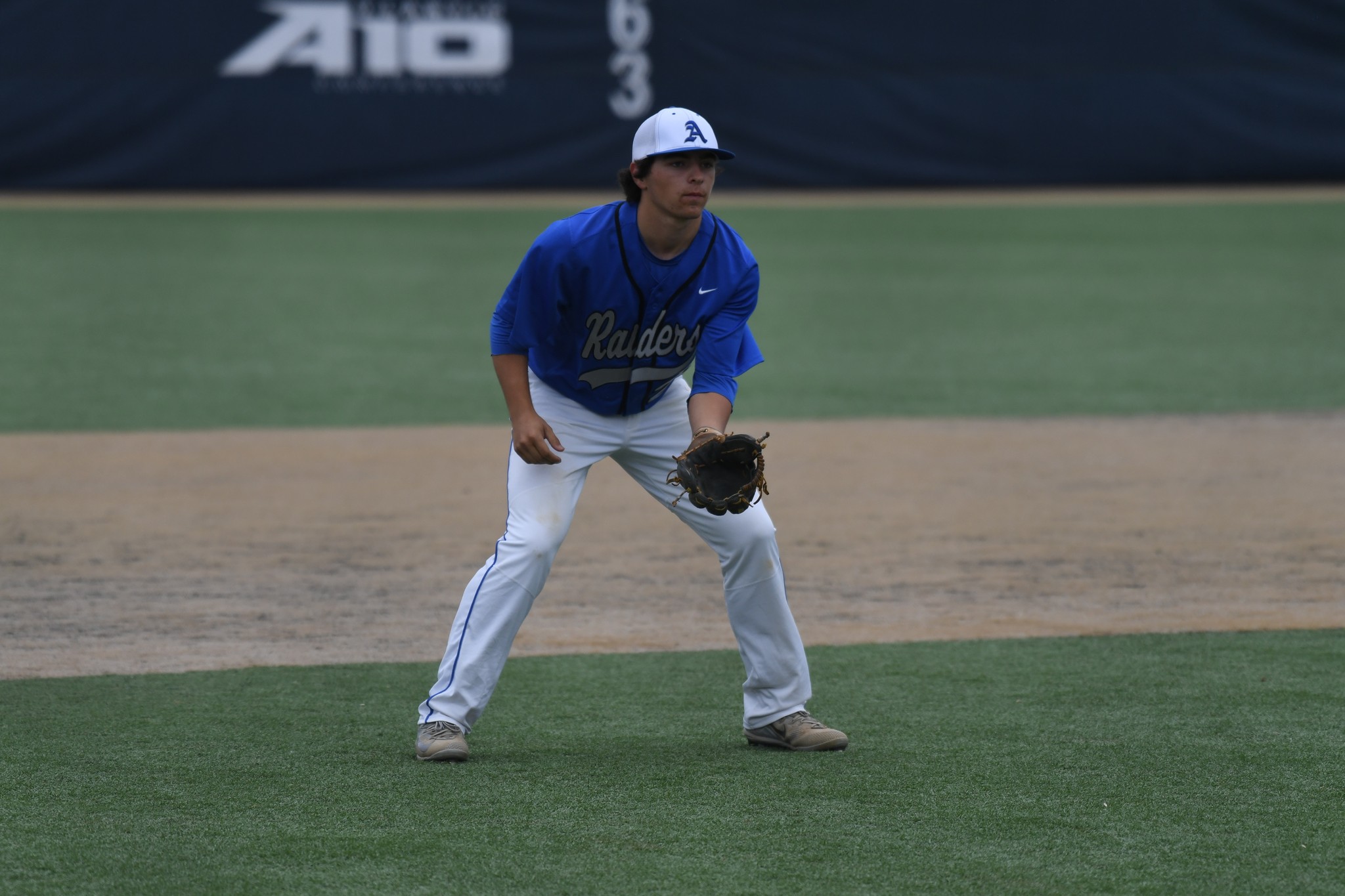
[742,709,848,750]
[414,720,469,762]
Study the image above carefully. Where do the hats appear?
[631,106,736,161]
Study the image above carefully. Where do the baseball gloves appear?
[670,432,766,516]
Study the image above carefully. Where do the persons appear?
[414,106,847,763]
